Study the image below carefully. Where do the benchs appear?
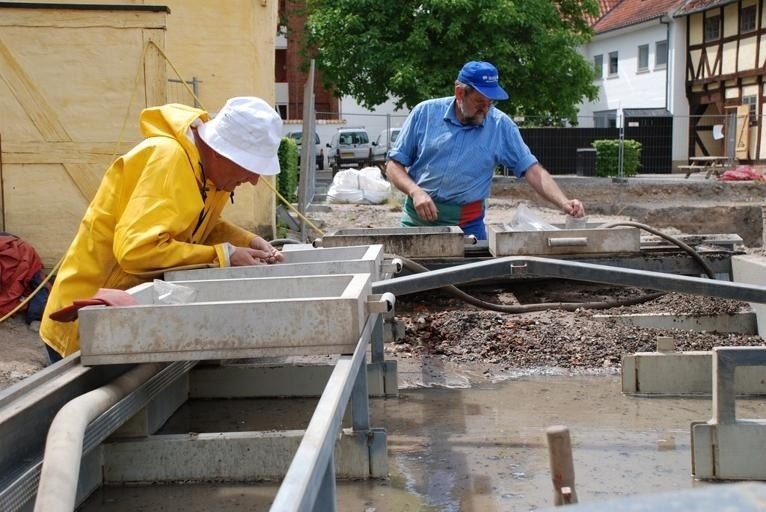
[677,164,737,180]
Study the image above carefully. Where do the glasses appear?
[468,95,499,111]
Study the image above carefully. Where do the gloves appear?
[50,288,137,322]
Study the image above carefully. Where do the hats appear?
[457,61,509,101]
[196,96,286,176]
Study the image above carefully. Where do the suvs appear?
[287,130,324,169]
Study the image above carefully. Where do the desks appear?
[683,156,732,179]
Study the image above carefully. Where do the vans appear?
[373,127,403,162]
[325,128,372,169]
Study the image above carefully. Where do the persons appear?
[385,59,585,239]
[40,94,284,365]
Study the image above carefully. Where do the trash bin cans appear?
[574,147,597,178]
